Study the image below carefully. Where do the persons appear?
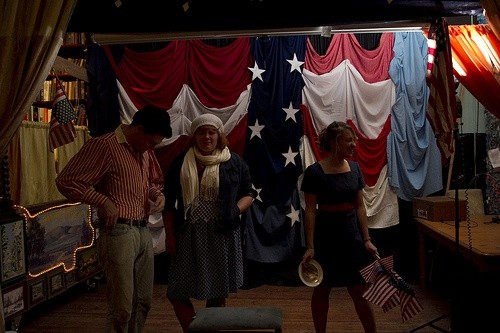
[55,106,172,333]
[157,113,253,333]
[302,121,379,333]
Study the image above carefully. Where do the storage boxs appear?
[412,196,466,222]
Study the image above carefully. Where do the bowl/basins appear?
[298,258,323,287]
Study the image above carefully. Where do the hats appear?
[191,113,224,137]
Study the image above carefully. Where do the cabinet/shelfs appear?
[18,32,89,126]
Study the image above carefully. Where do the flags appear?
[360,255,423,323]
[48,78,78,152]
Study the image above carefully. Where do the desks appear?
[415,215,500,289]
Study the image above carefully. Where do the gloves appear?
[214,203,241,233]
[173,211,187,239]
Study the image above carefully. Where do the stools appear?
[187,307,282,333]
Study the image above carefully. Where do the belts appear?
[117,216,148,228]
